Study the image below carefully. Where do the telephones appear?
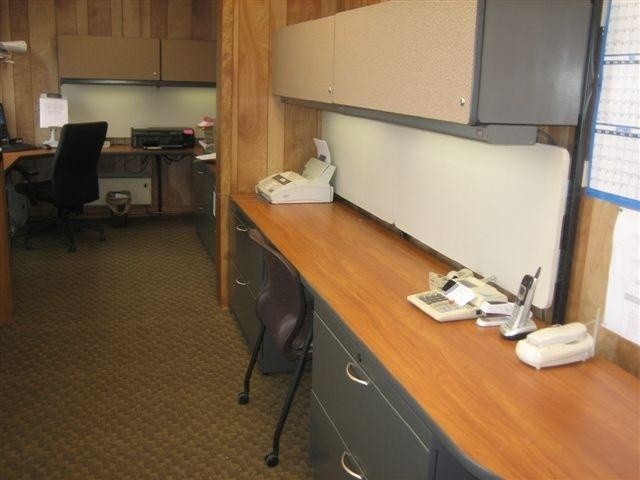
[500,267,542,340]
[515,322,594,369]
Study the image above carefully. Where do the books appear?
[196,114,217,164]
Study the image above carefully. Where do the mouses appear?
[39,145,50,150]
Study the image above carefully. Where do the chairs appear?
[12,122,110,249]
[239,228,316,465]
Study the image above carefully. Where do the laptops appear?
[0,103,38,153]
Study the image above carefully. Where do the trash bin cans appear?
[107,191,130,228]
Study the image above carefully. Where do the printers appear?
[255,157,336,204]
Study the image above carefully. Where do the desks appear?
[2,135,200,227]
[228,187,640,480]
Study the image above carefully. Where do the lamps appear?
[39,95,67,148]
[0,39,27,66]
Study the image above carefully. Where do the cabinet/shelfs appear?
[311,299,437,479]
[336,0,594,145]
[268,15,335,113]
[57,32,160,84]
[160,38,217,86]
[193,160,216,260]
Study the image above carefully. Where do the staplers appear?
[477,302,533,327]
